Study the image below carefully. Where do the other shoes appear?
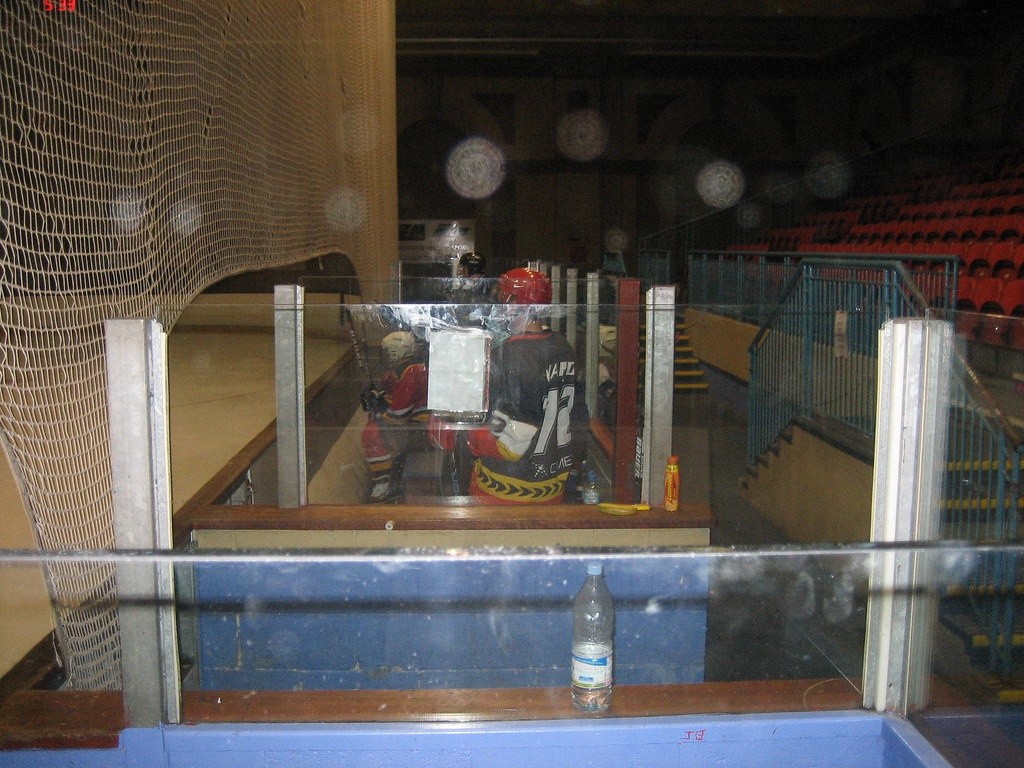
[369,470,402,503]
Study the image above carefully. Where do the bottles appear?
[577,461,600,505]
[664,456,679,511]
[570,562,613,712]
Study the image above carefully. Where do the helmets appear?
[497,268,553,303]
[457,252,486,278]
[380,331,414,369]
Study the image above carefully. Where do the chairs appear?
[720,152,1024,375]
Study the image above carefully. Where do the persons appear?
[361,253,582,505]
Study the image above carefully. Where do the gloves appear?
[361,386,380,411]
